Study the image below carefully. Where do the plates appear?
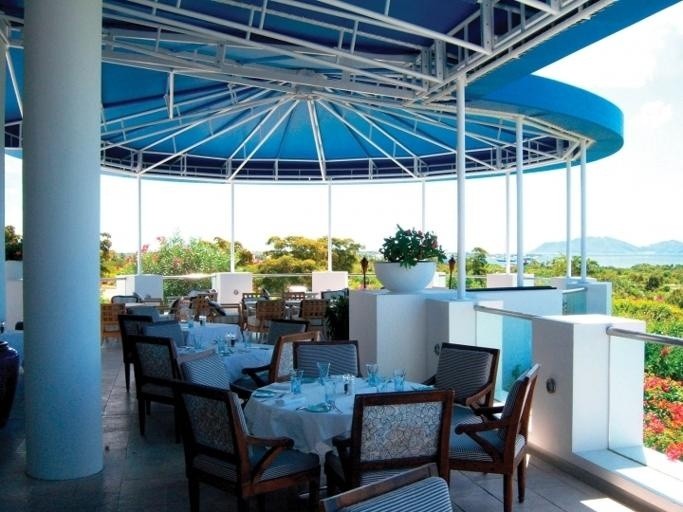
[252,390,276,398]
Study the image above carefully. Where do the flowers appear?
[379,224,448,270]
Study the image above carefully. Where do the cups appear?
[288,359,407,412]
[185,311,252,356]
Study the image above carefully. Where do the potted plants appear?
[5,225,23,280]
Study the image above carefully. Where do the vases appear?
[0,340,20,430]
[374,261,436,293]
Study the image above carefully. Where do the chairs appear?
[293,340,361,378]
[446,364,541,510]
[173,382,320,512]
[102,290,330,447]
[320,462,454,511]
[296,387,454,511]
[420,342,500,429]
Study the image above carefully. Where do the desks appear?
[242,373,434,511]
